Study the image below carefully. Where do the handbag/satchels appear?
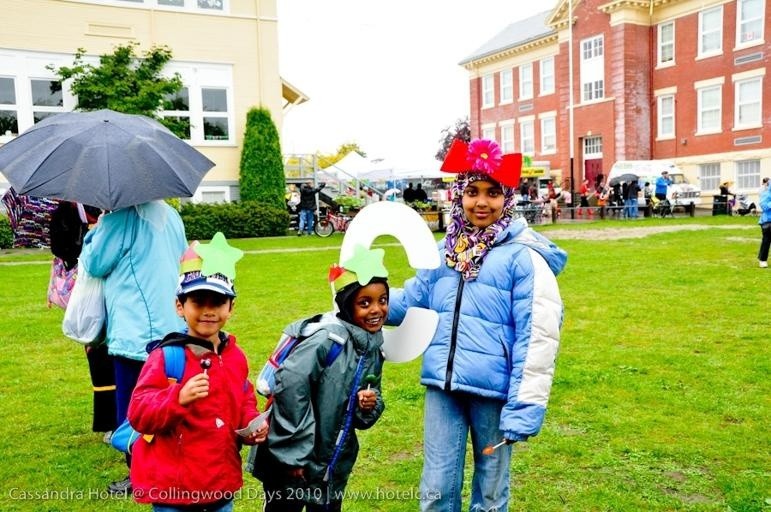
[48,254,79,310]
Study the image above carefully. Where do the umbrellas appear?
[0,105,218,204]
[1,181,81,252]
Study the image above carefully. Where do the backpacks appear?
[108,345,249,455]
[255,331,383,400]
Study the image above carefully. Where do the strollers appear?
[731,193,757,216]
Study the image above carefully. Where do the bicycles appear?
[651,192,685,219]
[314,207,354,238]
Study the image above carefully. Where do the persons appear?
[387,138,564,508]
[296,179,327,234]
[738,194,757,216]
[404,171,675,221]
[130,231,271,511]
[720,182,736,196]
[287,184,301,216]
[758,178,771,269]
[48,198,118,442]
[249,242,389,509]
[81,199,188,494]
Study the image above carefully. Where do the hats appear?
[176,271,236,298]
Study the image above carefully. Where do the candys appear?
[366,374,376,392]
[200,359,211,374]
[483,441,506,455]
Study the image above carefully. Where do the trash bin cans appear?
[441,209,452,230]
[712,194,728,216]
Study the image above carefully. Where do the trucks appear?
[604,160,700,213]
[513,175,562,205]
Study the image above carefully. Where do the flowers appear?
[465,140,504,174]
